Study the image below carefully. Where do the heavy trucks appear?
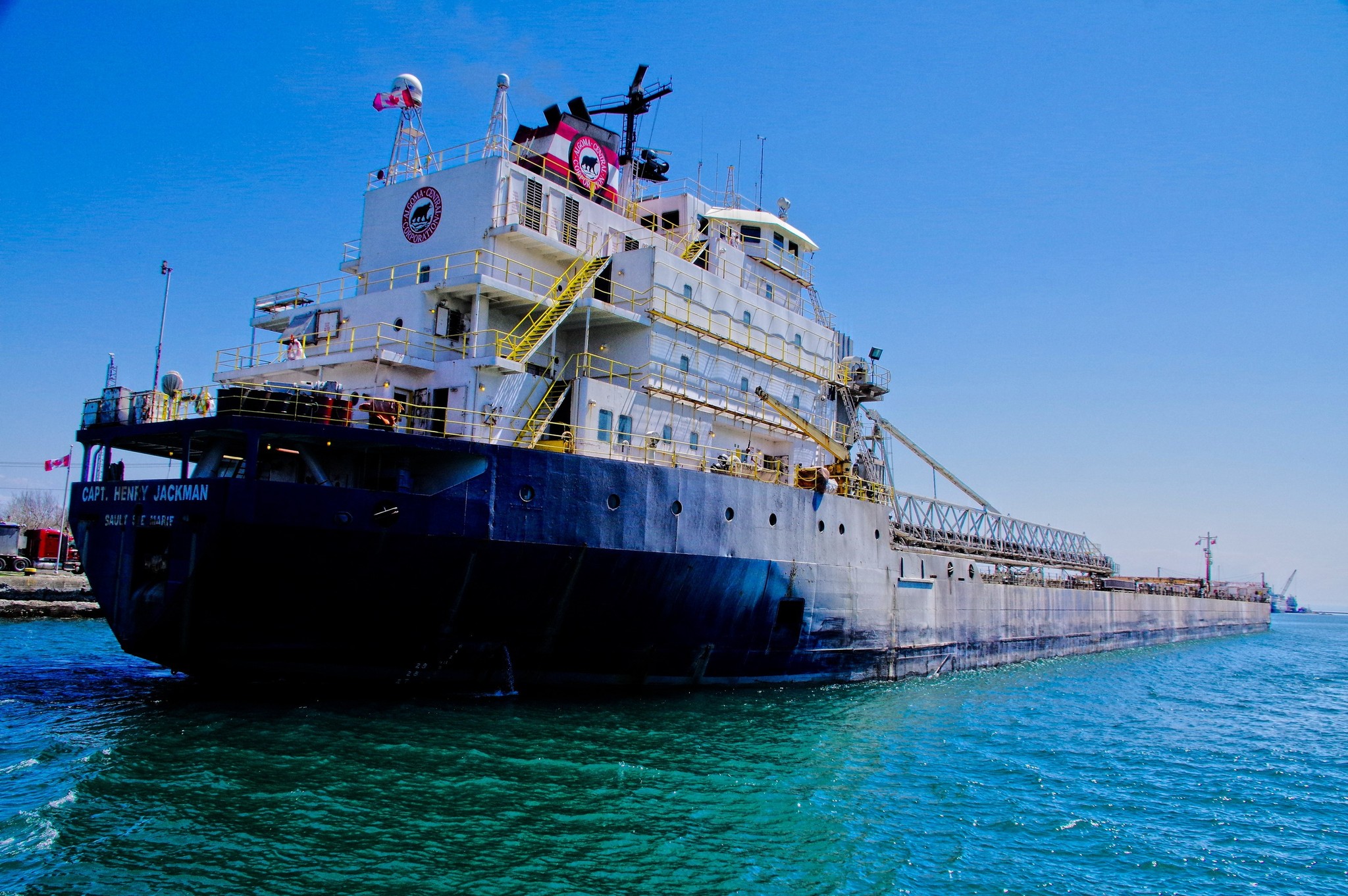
[0,516,84,575]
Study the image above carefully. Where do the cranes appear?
[1277,569,1297,598]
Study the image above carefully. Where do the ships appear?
[68,65,1272,691]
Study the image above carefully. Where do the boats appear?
[1286,594,1312,613]
[1269,593,1290,613]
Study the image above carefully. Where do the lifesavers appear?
[729,232,740,249]
[286,340,300,361]
[194,390,212,416]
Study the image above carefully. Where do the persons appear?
[1038,567,1043,573]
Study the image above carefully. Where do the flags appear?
[45,454,70,471]
[1203,548,1207,551]
[1195,540,1200,545]
[373,88,414,112]
[1211,539,1216,544]
